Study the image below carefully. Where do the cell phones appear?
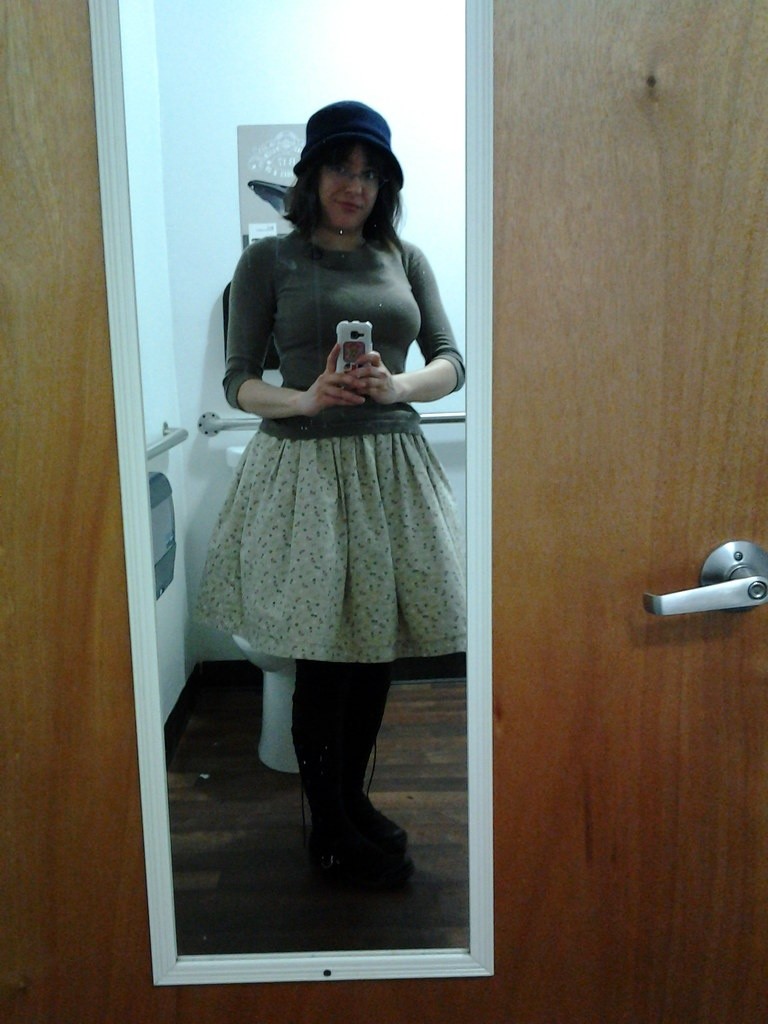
[337,320,373,388]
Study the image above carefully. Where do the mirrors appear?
[88,0,494,986]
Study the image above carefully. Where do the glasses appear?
[318,159,380,186]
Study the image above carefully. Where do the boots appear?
[292,707,415,893]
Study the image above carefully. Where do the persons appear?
[196,100,466,881]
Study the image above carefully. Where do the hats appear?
[293,101,403,192]
